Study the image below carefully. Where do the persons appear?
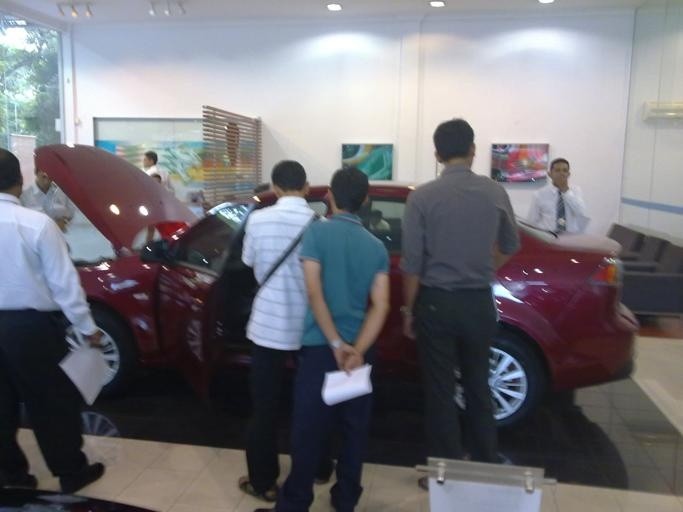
[235,158,329,504]
[252,164,396,512]
[17,164,76,234]
[0,146,105,496]
[397,116,525,490]
[140,149,158,176]
[367,209,391,232]
[150,173,162,183]
[524,157,593,236]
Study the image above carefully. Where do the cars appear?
[27,142,641,428]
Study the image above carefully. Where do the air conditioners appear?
[642,99,683,121]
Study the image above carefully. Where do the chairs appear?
[605,223,683,318]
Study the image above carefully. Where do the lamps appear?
[148,1,186,16]
[55,3,93,17]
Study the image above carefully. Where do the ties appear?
[555,190,566,231]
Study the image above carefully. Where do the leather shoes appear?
[59,462,104,494]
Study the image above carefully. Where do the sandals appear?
[238,475,279,502]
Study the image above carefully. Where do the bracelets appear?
[327,337,345,351]
[398,305,417,318]
